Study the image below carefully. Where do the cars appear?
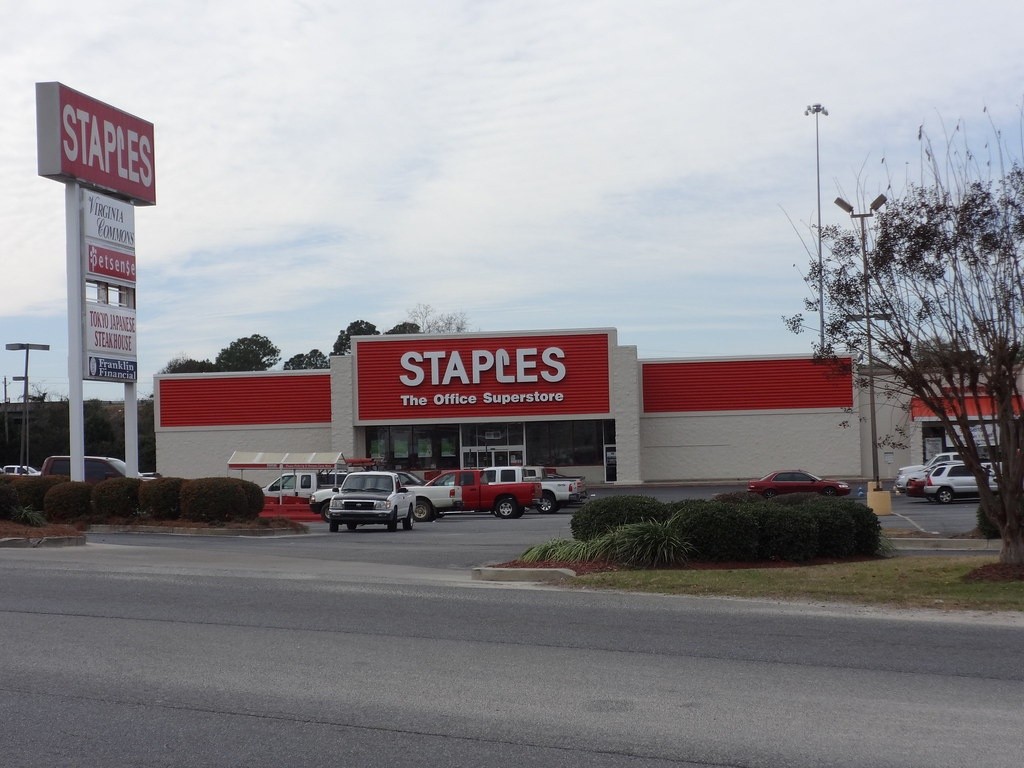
[390,471,426,487]
[747,468,852,501]
[893,451,999,506]
[0,464,41,477]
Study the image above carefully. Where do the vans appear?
[39,454,157,483]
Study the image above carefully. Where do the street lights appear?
[4,341,51,477]
[831,193,890,491]
[802,103,831,355]
[12,375,30,477]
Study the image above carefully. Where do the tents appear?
[227,451,347,506]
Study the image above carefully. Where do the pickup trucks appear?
[327,469,417,533]
[449,463,587,515]
[422,469,544,519]
[262,471,347,499]
[309,476,464,524]
